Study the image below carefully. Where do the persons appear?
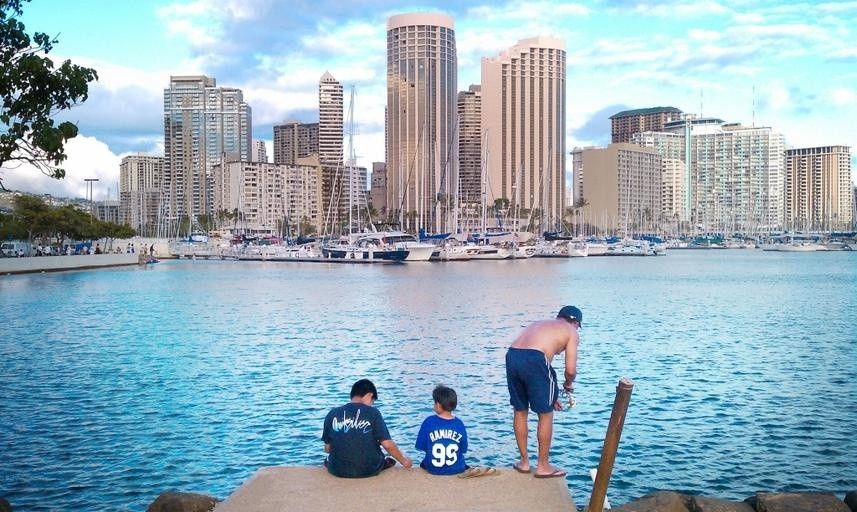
[505,304,582,479]
[415,385,469,475]
[15,243,154,256]
[322,380,413,478]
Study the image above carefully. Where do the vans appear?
[1,242,31,257]
[64,243,91,255]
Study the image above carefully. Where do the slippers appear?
[458,467,499,478]
[514,464,530,473]
[534,471,566,478]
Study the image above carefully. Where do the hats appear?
[558,306,583,328]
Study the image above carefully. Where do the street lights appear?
[84,177,100,225]
[45,194,52,204]
